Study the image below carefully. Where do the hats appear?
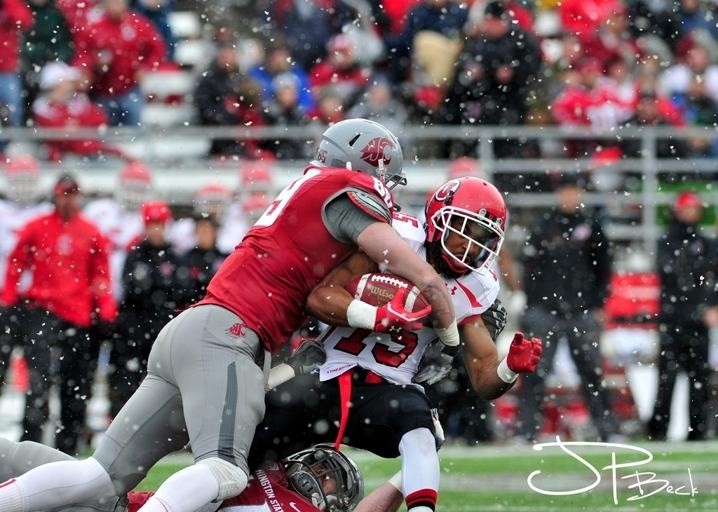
[142,200,171,222]
[54,176,78,194]
[672,192,702,211]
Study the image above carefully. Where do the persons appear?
[1,118,460,511]
[0,1,714,458]
[1,408,446,511]
[1,176,543,511]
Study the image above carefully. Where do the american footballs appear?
[345,273,432,326]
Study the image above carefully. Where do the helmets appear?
[425,176,506,274]
[284,443,364,511]
[316,118,407,190]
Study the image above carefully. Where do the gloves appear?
[481,299,507,340]
[285,338,327,377]
[430,408,445,452]
[373,288,432,336]
[507,332,544,374]
[411,337,463,385]
